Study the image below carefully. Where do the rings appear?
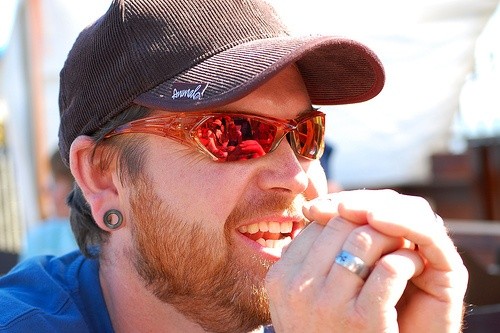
[334,251,371,283]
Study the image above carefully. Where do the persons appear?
[0,0,468,333]
[23,151,80,259]
[196,116,324,160]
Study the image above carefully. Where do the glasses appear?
[96,109,327,164]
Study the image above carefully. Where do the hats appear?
[57,0,386,170]
